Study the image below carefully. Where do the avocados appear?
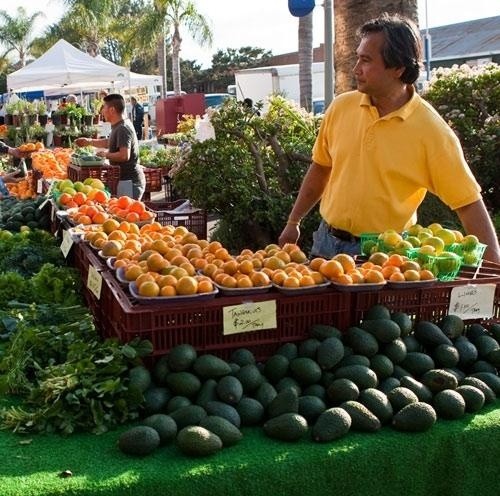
[116,305,500,457]
[0,197,49,232]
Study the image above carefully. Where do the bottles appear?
[0,177,9,196]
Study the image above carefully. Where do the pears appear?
[57,178,105,197]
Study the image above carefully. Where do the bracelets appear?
[286,220,301,225]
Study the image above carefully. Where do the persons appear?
[130,96,145,141]
[0,140,26,183]
[74,94,146,202]
[277,10,499,323]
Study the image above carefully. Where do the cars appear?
[147,86,238,122]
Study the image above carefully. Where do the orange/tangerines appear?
[84,220,479,297]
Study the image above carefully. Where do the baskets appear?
[31,162,207,264]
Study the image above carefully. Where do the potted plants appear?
[0,99,104,147]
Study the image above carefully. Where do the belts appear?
[320,219,360,243]
